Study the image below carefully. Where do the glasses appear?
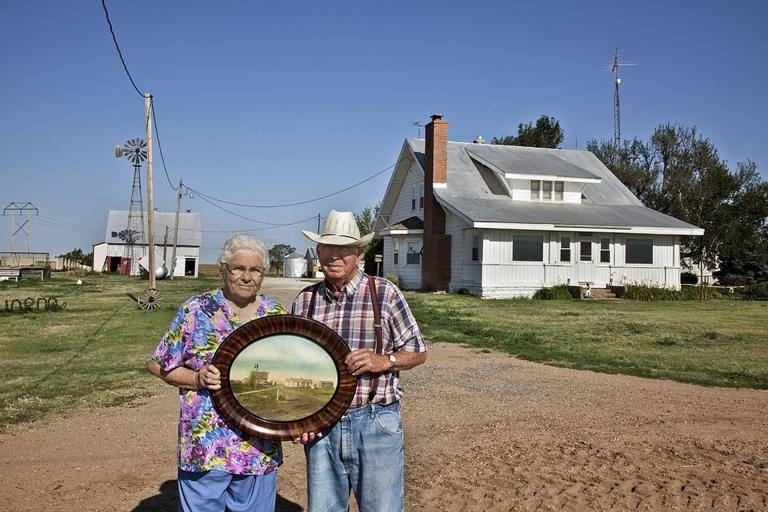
[225,262,265,278]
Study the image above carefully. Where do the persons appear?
[291,209,427,512]
[147,234,291,512]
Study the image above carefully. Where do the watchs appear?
[384,351,397,373]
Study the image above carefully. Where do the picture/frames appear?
[212,314,357,441]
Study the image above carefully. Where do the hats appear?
[301,210,375,248]
